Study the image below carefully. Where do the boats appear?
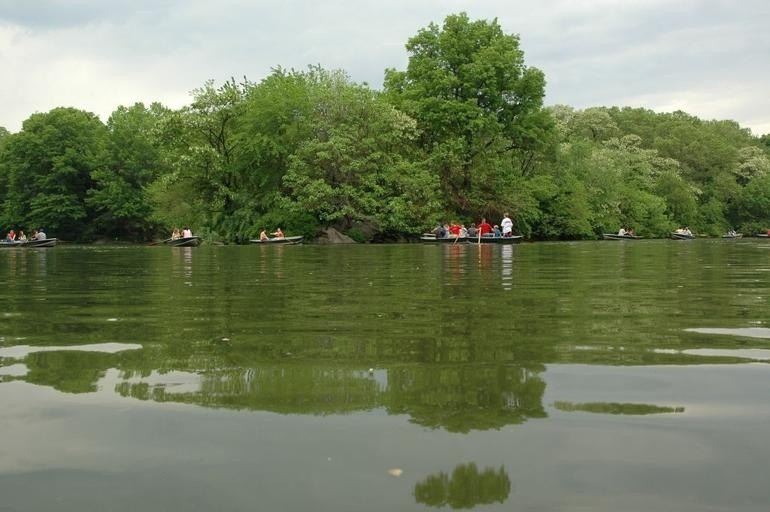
[249,235,305,244]
[0,238,57,247]
[722,229,770,238]
[420,233,523,243]
[671,233,696,240]
[149,236,202,247]
[603,234,644,240]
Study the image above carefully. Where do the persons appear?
[172,227,192,238]
[7,228,46,241]
[676,227,692,236]
[430,212,513,238]
[260,228,284,240]
[619,225,635,236]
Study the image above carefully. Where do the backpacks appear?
[436,225,446,238]
